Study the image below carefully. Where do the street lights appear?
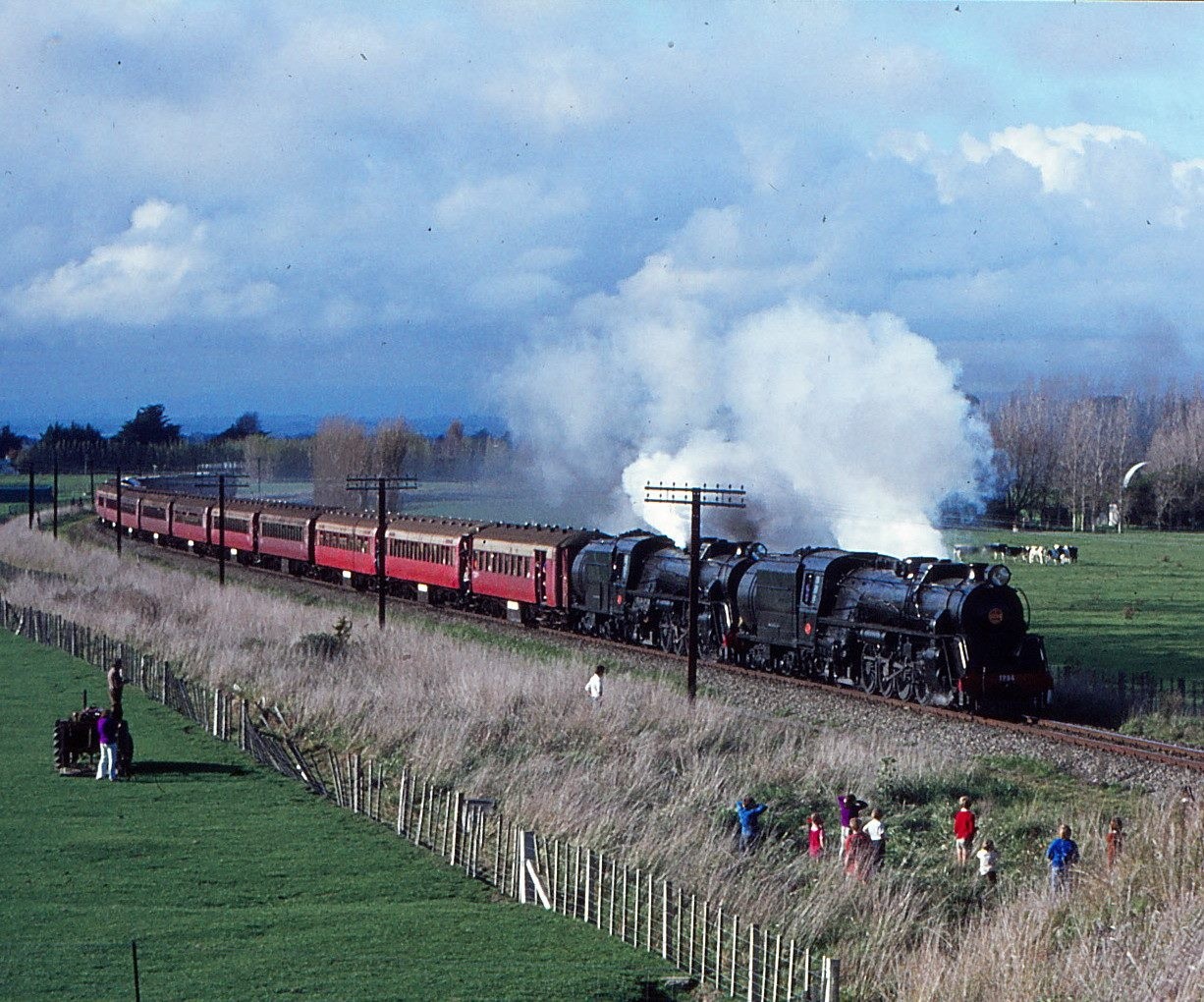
[343,470,419,633]
[191,470,248,588]
[641,478,748,713]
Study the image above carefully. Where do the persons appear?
[118,721,133,776]
[863,808,889,869]
[734,797,768,856]
[96,710,119,782]
[1047,825,1079,890]
[107,658,127,720]
[584,666,605,709]
[1107,817,1127,869]
[844,818,870,880]
[838,795,867,859]
[954,796,976,868]
[976,840,1000,887]
[807,813,825,859]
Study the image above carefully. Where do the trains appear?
[90,467,1058,723]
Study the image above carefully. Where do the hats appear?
[744,798,754,805]
[111,659,122,666]
[1111,817,1122,829]
[850,818,862,827]
[102,710,110,716]
[959,795,970,805]
[871,807,882,817]
[982,839,993,848]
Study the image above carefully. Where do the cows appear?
[1046,542,1079,566]
[1005,546,1031,564]
[950,544,982,562]
[981,543,1009,564]
[1021,544,1050,565]
